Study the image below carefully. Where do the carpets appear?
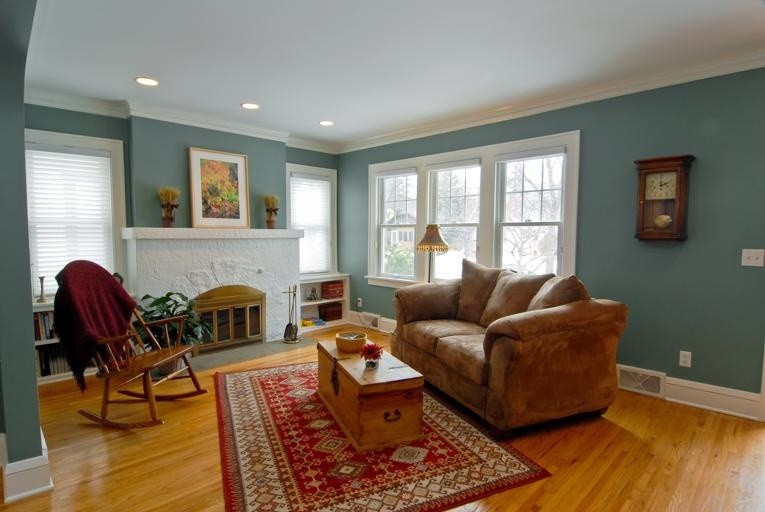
[214,361,553,512]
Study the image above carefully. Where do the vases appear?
[364,357,383,370]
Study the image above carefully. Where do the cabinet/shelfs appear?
[299,273,351,333]
[32,297,99,385]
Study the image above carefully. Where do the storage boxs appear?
[321,283,343,300]
[319,302,342,322]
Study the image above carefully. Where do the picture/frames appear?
[188,147,251,230]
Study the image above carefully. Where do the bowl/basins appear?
[335,330,367,352]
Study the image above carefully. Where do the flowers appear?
[360,345,383,360]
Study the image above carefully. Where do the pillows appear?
[480,269,555,329]
[457,258,507,322]
[526,276,591,311]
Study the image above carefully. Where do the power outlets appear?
[678,351,692,367]
[357,299,362,308]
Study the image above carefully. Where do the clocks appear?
[634,155,693,243]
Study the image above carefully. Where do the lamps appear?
[417,224,449,283]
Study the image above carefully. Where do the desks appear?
[315,340,424,453]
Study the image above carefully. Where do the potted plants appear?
[263,196,281,229]
[158,189,180,227]
[128,292,213,376]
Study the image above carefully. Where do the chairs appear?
[56,259,208,431]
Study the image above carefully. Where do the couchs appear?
[390,277,627,439]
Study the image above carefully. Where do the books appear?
[301,317,325,328]
[34,311,57,341]
[36,344,96,377]
